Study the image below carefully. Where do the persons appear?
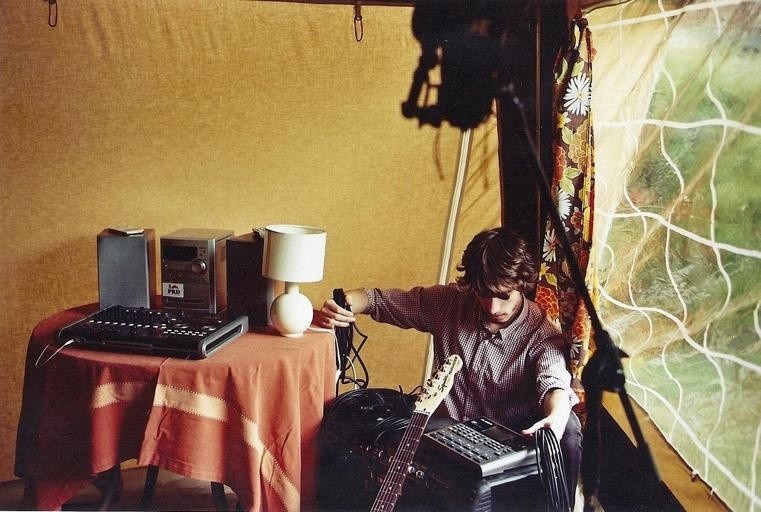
[315,224,585,512]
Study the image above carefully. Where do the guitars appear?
[369,353,463,511]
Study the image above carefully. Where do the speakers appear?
[225,232,274,328]
[97,228,156,308]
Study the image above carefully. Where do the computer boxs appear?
[160,228,235,317]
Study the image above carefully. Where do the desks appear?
[14,296,337,511]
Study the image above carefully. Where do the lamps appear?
[261,223,327,338]
